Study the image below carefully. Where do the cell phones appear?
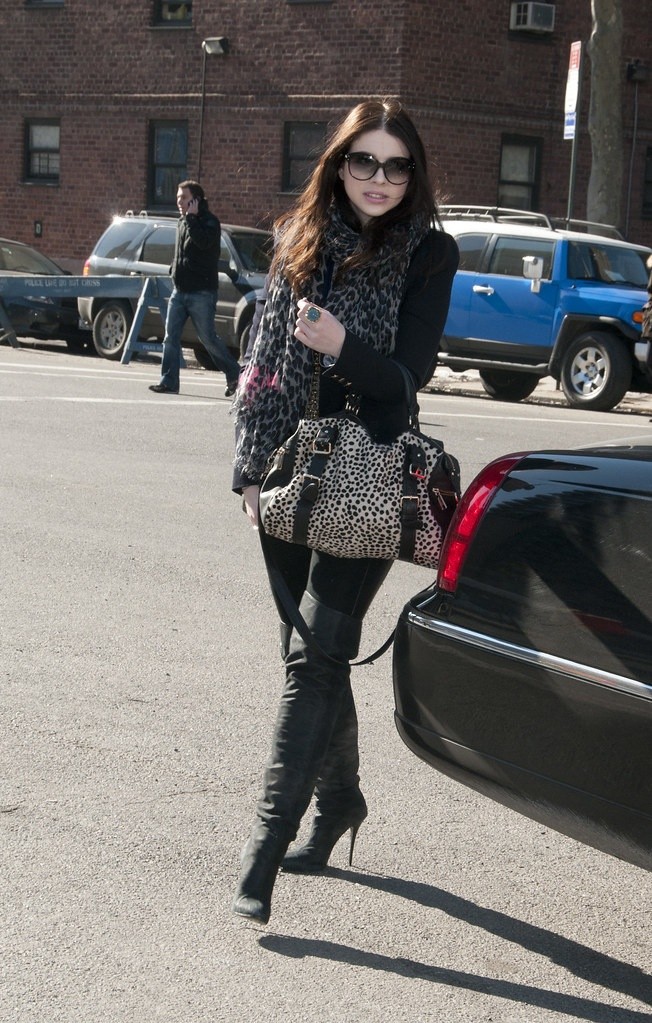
[188,195,201,206]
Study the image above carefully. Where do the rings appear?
[305,306,323,324]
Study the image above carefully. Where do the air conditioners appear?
[510,1,555,33]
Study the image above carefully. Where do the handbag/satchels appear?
[258,360,461,569]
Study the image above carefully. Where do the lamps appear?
[203,37,228,55]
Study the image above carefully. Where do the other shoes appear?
[149,383,179,394]
[224,378,239,397]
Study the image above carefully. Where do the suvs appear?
[431,202,652,412]
[75,207,276,372]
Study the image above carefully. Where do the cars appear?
[0,235,94,350]
[391,443,652,874]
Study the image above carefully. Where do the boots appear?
[232,591,362,927]
[279,621,368,874]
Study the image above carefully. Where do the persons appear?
[149,180,241,397]
[234,101,460,924]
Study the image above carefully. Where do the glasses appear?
[337,151,415,185]
